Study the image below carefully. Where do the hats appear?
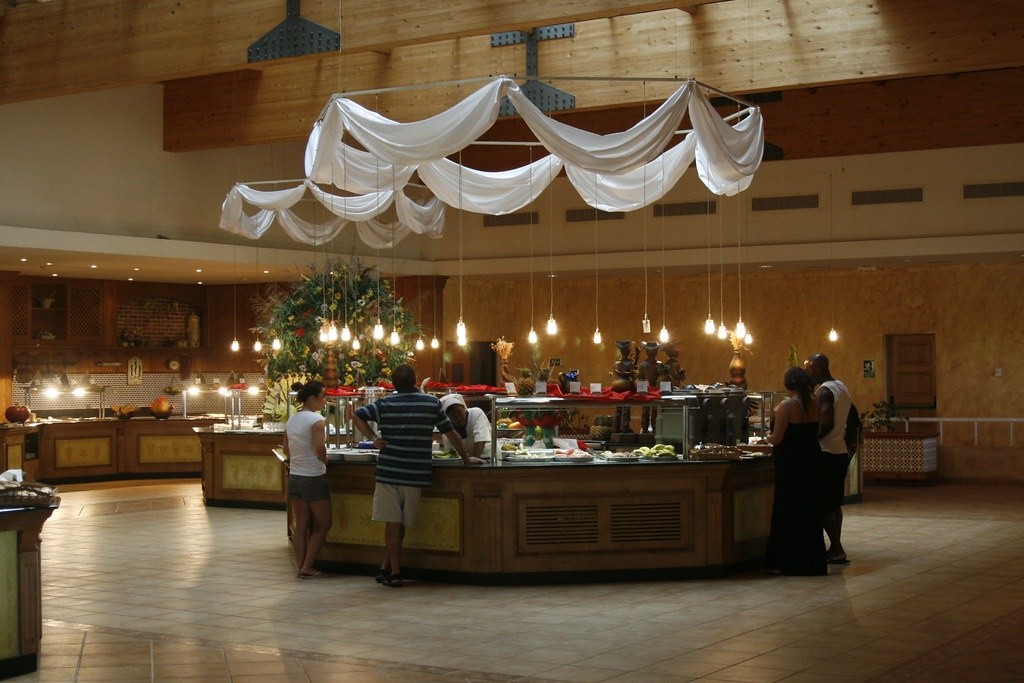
[439,394,467,413]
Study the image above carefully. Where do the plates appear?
[167,391,180,395]
[505,449,594,461]
[33,334,56,339]
[672,389,746,394]
[607,455,678,461]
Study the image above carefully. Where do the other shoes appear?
[827,551,851,564]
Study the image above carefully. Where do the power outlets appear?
[995,367,1001,377]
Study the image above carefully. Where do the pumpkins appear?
[5,401,32,424]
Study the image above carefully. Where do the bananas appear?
[433,448,459,458]
[633,444,678,458]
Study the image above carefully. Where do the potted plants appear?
[859,400,909,433]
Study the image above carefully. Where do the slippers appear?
[296,569,325,578]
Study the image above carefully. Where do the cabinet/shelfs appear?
[9,274,304,376]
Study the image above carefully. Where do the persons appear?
[766,367,828,577]
[439,394,502,460]
[353,363,486,588]
[283,380,330,579]
[612,339,686,433]
[803,353,851,563]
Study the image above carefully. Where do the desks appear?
[863,425,940,487]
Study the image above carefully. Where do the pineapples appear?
[590,415,613,440]
[517,368,534,397]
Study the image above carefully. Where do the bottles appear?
[178,335,188,348]
[186,312,201,347]
[325,345,338,388]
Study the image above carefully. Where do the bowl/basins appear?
[683,384,696,389]
[603,443,655,452]
[151,412,172,419]
[501,451,516,459]
[497,428,524,438]
[37,297,55,308]
[748,437,766,444]
[114,413,133,419]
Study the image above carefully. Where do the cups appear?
[31,413,36,422]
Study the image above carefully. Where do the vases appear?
[729,350,747,386]
[500,359,509,388]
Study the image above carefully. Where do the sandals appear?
[382,574,402,587]
[376,570,391,582]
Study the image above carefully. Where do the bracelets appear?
[369,433,378,442]
[767,435,770,439]
[462,453,469,460]
